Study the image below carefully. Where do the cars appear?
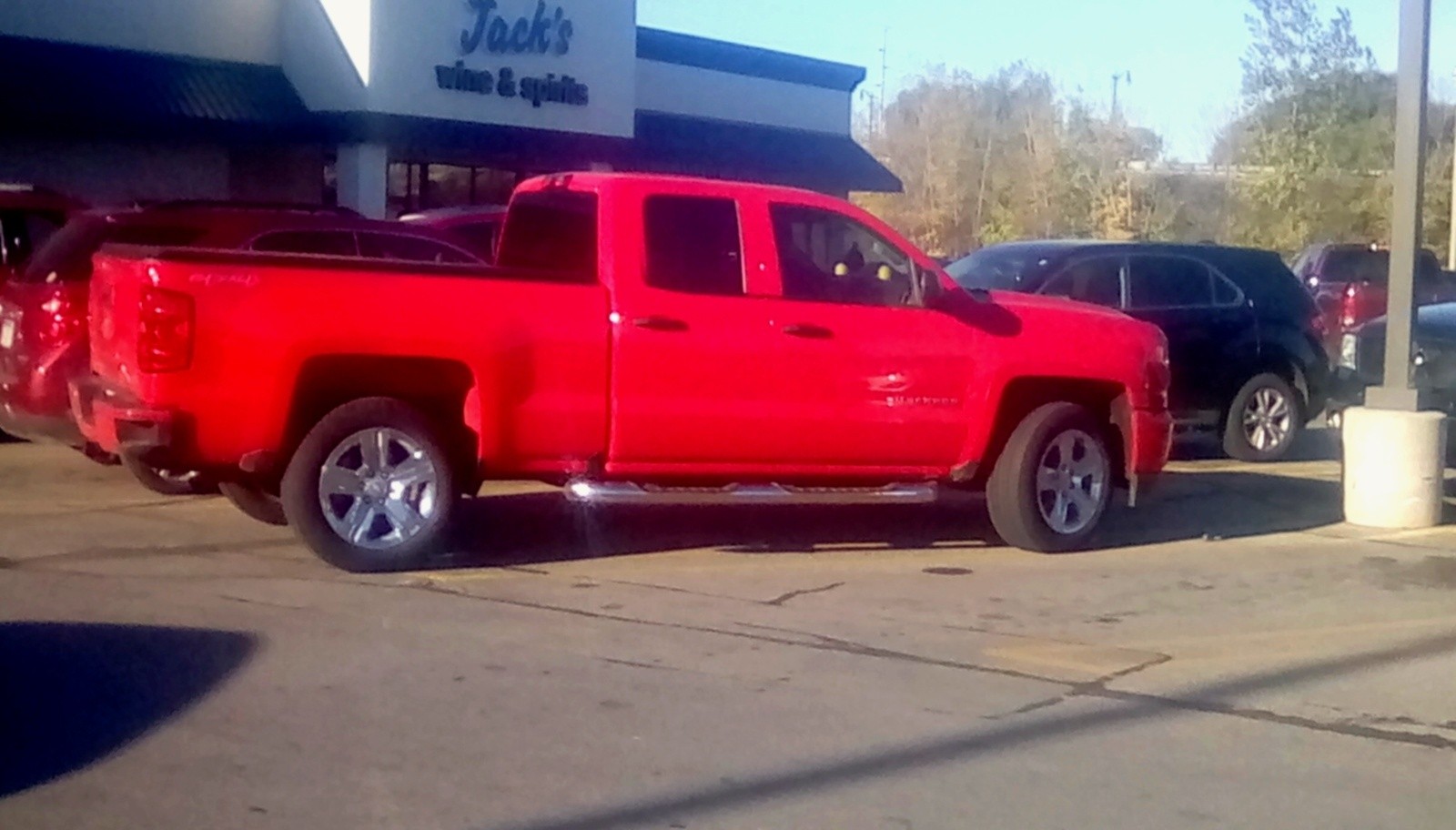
[395,201,682,285]
[1322,300,1456,467]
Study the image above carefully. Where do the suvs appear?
[0,194,496,498]
[902,236,1336,466]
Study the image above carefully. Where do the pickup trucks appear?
[64,167,1177,575]
[1283,240,1456,379]
